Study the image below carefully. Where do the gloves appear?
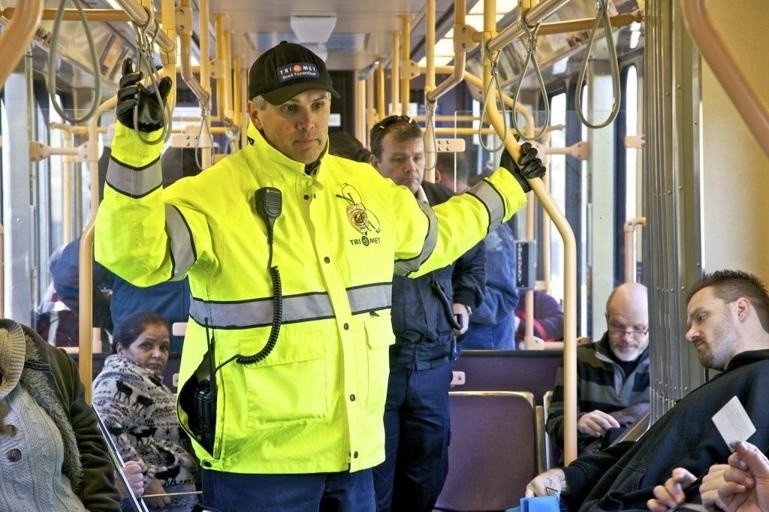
[500,134,545,193]
[117,57,172,132]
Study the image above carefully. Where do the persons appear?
[545,283,650,465]
[39,243,112,355]
[514,288,564,348]
[51,150,203,393]
[328,130,371,163]
[0,319,123,512]
[91,309,203,512]
[507,268,769,512]
[94,40,546,512]
[368,115,486,512]
[646,464,732,512]
[434,153,520,353]
[718,440,769,512]
[113,461,145,499]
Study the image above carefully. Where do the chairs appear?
[438,381,545,511]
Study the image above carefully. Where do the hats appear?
[249,41,341,105]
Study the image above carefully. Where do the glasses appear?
[375,116,418,131]
